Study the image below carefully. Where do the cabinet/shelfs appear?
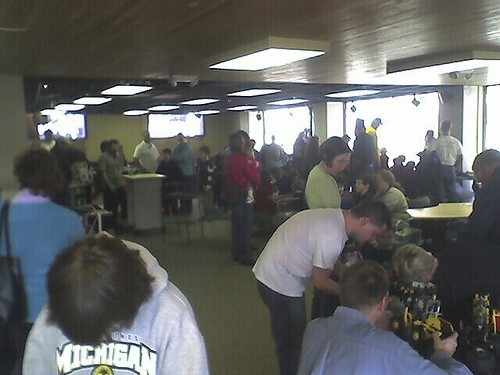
[123,173,165,235]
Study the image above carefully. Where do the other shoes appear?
[234,256,256,266]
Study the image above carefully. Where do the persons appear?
[42,129,198,223]
[0,142,88,336]
[22,231,210,375]
[202,117,500,375]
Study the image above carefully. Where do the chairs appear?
[160,174,204,247]
[88,210,113,233]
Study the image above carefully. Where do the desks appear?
[406,202,475,254]
[265,193,301,233]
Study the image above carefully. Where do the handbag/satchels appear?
[0,255,29,325]
[219,190,243,209]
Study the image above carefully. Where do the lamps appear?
[386,48,500,79]
[207,36,330,73]
